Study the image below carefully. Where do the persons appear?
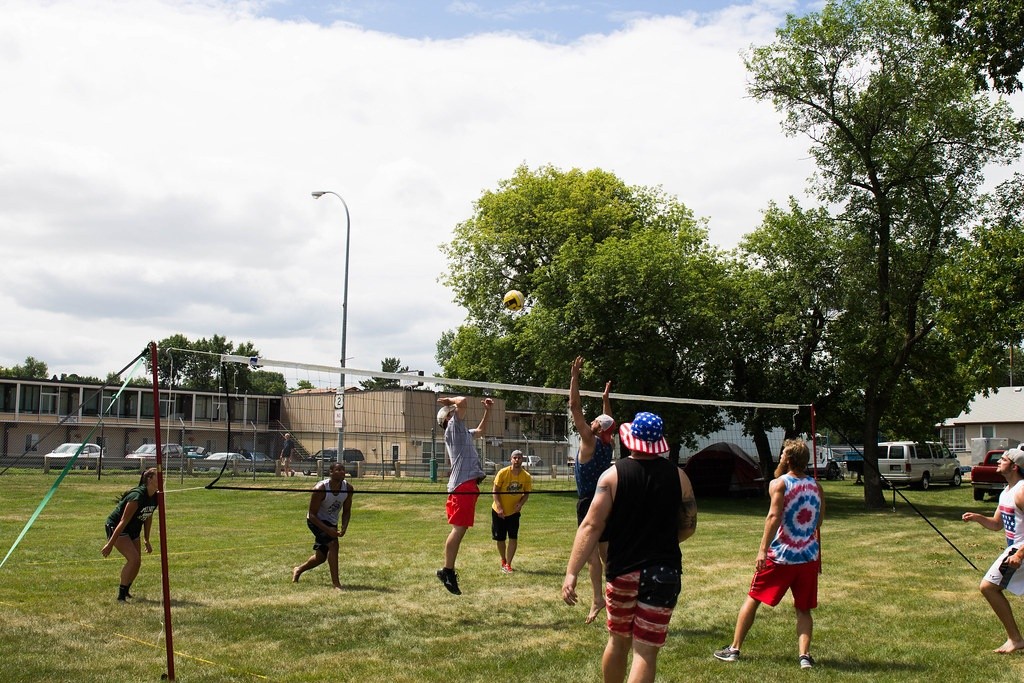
[569,356,615,623]
[437,395,494,595]
[293,462,354,591]
[561,412,697,683]
[714,439,825,671]
[280,434,295,478]
[101,467,159,604]
[491,450,531,574]
[962,448,1024,653]
[239,446,252,472]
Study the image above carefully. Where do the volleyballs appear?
[504,290,525,311]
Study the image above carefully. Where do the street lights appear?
[313,189,351,464]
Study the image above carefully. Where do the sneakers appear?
[799,654,816,670]
[714,645,740,662]
[437,567,462,595]
[501,558,507,570]
[504,564,513,573]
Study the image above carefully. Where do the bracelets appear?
[1015,550,1024,559]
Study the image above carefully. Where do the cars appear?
[480,458,496,475]
[241,452,276,472]
[199,452,252,470]
[45,443,104,470]
[184,446,208,459]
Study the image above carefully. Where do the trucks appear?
[610,410,842,481]
[836,450,864,474]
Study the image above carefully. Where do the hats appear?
[511,450,523,459]
[1006,448,1024,468]
[595,413,617,445]
[619,410,670,453]
[437,403,457,427]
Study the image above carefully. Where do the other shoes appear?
[291,470,295,477]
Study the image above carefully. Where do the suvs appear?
[521,455,544,474]
[878,440,964,490]
[125,443,182,470]
[303,447,365,478]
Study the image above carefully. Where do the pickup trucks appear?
[971,450,1008,501]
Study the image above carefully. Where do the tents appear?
[685,442,769,500]
